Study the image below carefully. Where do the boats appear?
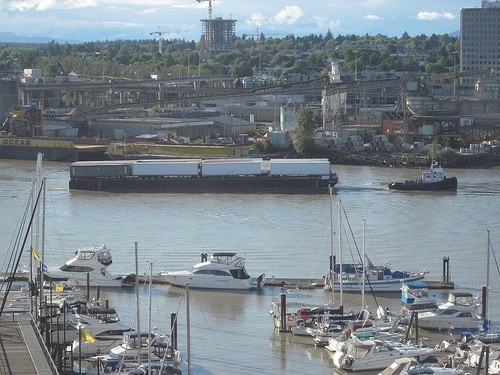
[269,300,360,332]
[322,253,430,293]
[288,305,401,336]
[305,311,412,348]
[69,158,339,194]
[89,332,183,372]
[400,283,442,311]
[387,159,457,191]
[377,357,465,375]
[410,292,491,332]
[128,364,182,375]
[65,327,136,359]
[333,335,434,371]
[158,252,266,290]
[434,324,500,375]
[325,324,429,352]
[36,281,121,345]
[0,109,107,162]
[39,244,136,287]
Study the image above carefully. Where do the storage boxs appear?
[69,157,331,178]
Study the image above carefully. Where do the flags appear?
[52,281,62,292]
[32,248,39,260]
[81,330,93,342]
